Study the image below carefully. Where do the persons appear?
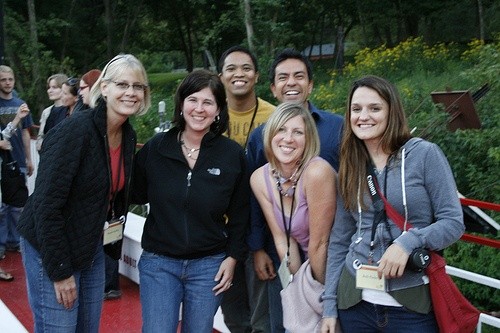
[78,69,130,299]
[18,53,151,333]
[35,73,68,149]
[1,101,30,282]
[134,70,246,333]
[249,102,339,333]
[246,48,345,332]
[319,75,465,333]
[0,64,35,260]
[60,76,81,116]
[216,46,277,321]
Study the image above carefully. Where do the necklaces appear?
[180,136,200,160]
[271,167,298,198]
[224,96,259,150]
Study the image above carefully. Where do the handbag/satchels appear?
[426,252,480,333]
[0,160,29,206]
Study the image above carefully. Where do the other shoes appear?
[0,248,5,258]
[0,271,14,281]
[104,285,121,297]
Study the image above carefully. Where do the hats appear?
[82,70,102,90]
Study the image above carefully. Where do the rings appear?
[228,282,232,286]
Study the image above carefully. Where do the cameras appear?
[387,240,430,273]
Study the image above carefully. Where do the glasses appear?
[80,86,89,90]
[67,79,76,86]
[111,80,148,90]
